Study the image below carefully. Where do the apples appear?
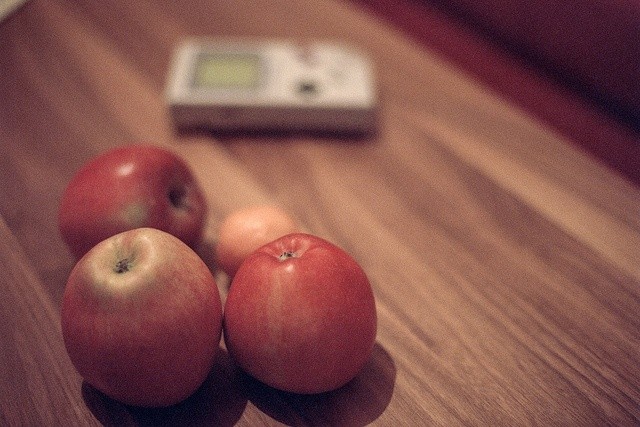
[223,233,377,392]
[59,146,205,249]
[62,227,223,406]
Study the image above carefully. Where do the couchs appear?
[358,0,639,188]
